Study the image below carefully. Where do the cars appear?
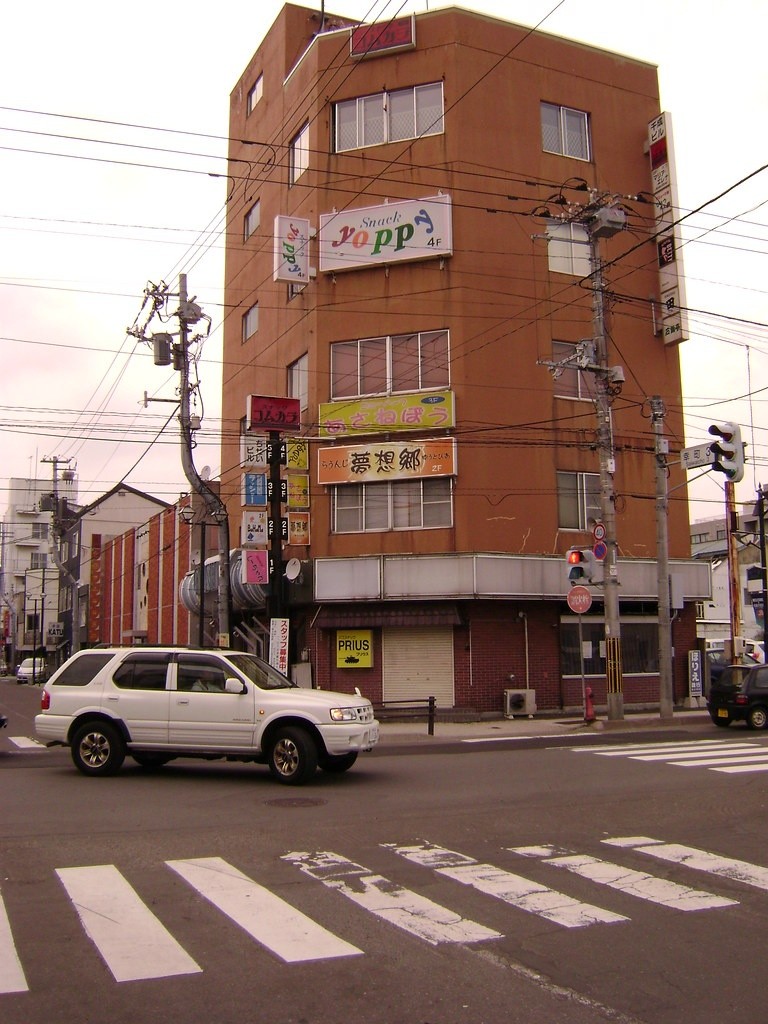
[705,637,765,665]
[708,663,768,729]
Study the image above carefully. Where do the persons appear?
[191,668,224,692]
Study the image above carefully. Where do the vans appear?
[17,657,50,684]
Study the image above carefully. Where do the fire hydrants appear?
[585,686,596,722]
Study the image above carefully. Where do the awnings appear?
[18,640,70,652]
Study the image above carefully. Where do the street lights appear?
[26,591,47,685]
[0,592,16,676]
[178,504,229,648]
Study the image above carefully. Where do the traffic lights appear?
[708,422,744,483]
[566,549,584,581]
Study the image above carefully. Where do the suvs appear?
[34,643,380,786]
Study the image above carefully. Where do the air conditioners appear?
[504,689,535,716]
